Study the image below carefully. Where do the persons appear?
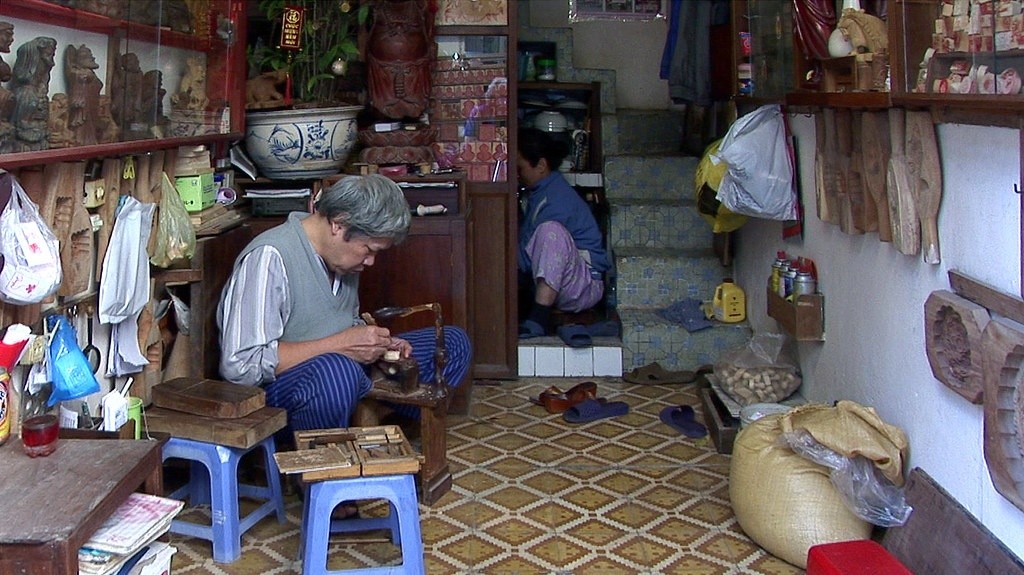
[64,44,103,145]
[49,93,77,147]
[518,127,610,339]
[216,174,471,519]
[10,36,57,121]
[111,52,143,128]
[0,21,16,121]
[356,0,437,121]
[98,95,120,143]
[142,69,170,124]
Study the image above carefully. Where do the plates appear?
[518,93,589,109]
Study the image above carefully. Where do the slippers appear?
[558,324,594,348]
[660,404,707,438]
[518,320,547,338]
[624,362,696,385]
[588,318,619,336]
[295,483,360,520]
[562,398,628,423]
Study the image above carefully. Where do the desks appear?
[0,415,170,575]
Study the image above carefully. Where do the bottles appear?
[537,59,555,80]
[872,48,888,90]
[784,259,799,306]
[792,266,816,307]
[526,54,535,81]
[712,278,746,322]
[771,250,787,294]
[778,260,791,302]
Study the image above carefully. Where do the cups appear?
[858,62,872,91]
[17,413,60,456]
[126,397,144,439]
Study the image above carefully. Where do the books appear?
[78,492,186,575]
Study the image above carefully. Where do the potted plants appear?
[244,0,371,179]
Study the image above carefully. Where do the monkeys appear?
[170,56,210,122]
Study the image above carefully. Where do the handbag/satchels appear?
[46,316,100,408]
[151,172,195,269]
[711,100,797,221]
[695,137,752,234]
[98,192,156,336]
[0,176,64,306]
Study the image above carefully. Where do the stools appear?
[157,434,285,562]
[301,475,426,575]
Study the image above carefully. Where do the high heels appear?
[528,382,596,405]
[545,390,607,413]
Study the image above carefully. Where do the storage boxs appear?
[701,387,737,454]
[173,172,216,213]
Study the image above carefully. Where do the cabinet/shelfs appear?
[153,0,598,414]
[0,0,247,167]
[730,0,1024,111]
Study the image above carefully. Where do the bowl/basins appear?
[533,111,568,132]
